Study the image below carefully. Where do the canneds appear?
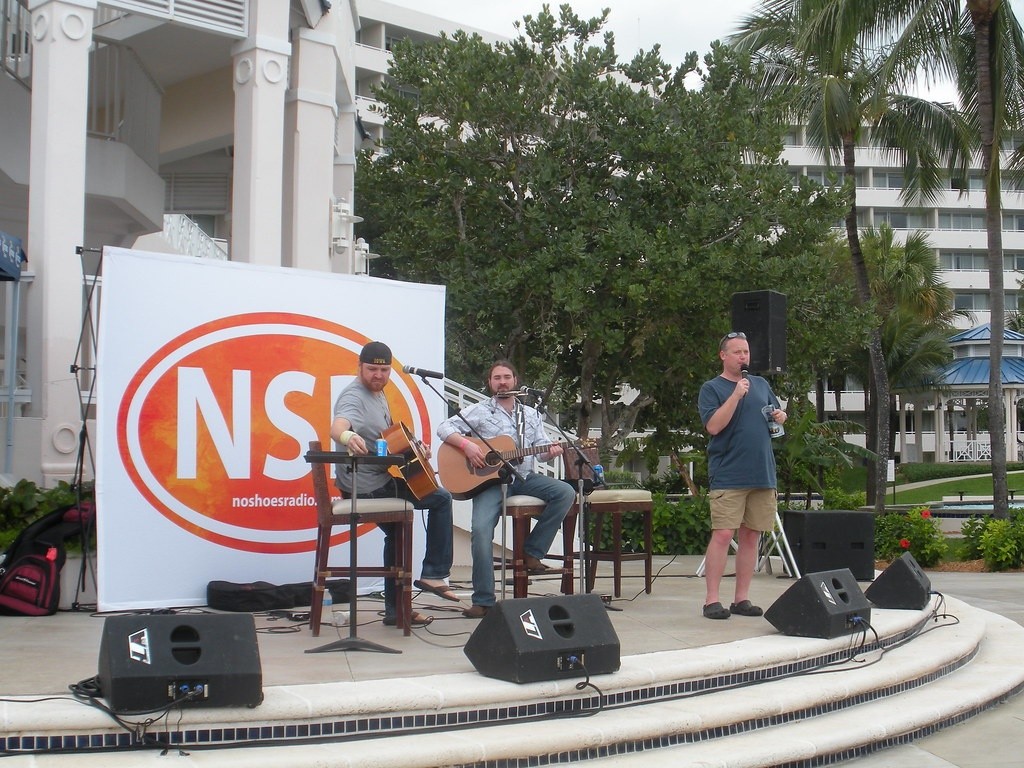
[593,465,604,483]
[375,438,388,457]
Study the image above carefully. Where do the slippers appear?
[414,579,460,602]
[383,610,431,625]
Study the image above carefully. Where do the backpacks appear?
[0,553,61,618]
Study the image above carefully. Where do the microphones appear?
[402,365,444,381]
[521,386,545,397]
[741,363,749,382]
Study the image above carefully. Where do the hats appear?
[360,341,392,365]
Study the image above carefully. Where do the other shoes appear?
[524,552,546,573]
[463,604,492,618]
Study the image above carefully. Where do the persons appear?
[436,361,576,617]
[697,332,787,619]
[330,342,460,624]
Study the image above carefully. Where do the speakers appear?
[463,593,621,683]
[763,567,871,640]
[730,290,788,377]
[864,552,932,610]
[98,614,264,713]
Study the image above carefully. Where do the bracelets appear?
[460,439,468,450]
[340,431,365,456]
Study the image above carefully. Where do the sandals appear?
[730,600,763,616]
[703,602,731,619]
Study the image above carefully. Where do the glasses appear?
[720,332,746,348]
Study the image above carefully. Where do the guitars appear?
[380,420,439,501]
[436,435,599,501]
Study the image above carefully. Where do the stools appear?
[499,490,583,599]
[308,442,414,636]
[560,448,654,597]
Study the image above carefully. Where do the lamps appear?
[327,195,381,276]
